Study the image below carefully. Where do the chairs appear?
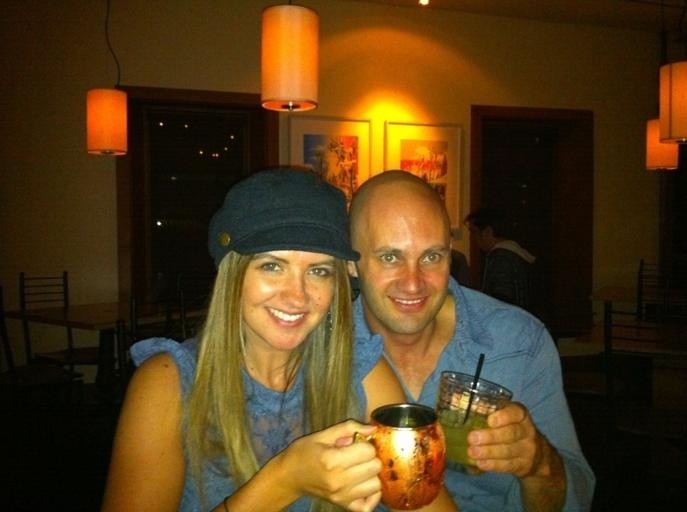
[552,247,687,491]
[1,260,218,420]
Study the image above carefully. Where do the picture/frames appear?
[382,116,466,237]
[283,106,374,222]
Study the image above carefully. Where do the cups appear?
[349,403,446,511]
[435,370,513,475]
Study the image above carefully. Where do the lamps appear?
[255,0,325,116]
[79,0,130,161]
[645,113,682,173]
[655,56,686,146]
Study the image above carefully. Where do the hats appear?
[207,168,360,275]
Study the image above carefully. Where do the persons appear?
[96,165,457,511]
[463,201,539,313]
[343,168,600,511]
